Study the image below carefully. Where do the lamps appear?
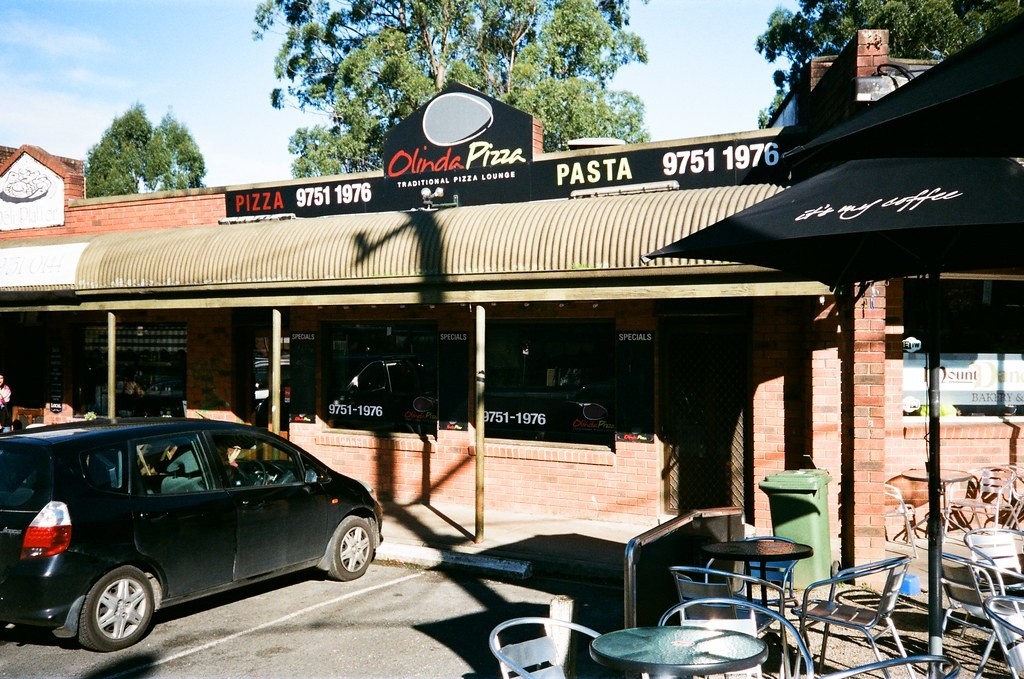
[420,186,465,210]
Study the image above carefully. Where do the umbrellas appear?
[647,158,1024,679]
[782,12,1023,168]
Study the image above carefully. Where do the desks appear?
[695,542,814,677]
[589,625,770,679]
[901,467,973,542]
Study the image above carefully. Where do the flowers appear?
[83,411,96,420]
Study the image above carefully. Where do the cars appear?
[250,353,297,406]
[145,380,187,399]
[327,353,435,435]
[0,419,383,652]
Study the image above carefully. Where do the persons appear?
[0,374,11,425]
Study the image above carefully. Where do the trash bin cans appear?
[758,468,842,590]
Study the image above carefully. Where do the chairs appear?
[489,455,1024,679]
[160,451,203,493]
